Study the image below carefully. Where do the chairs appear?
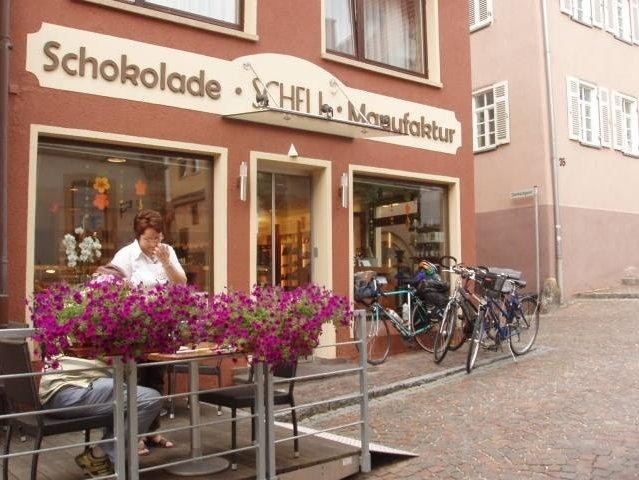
[168,357,222,419]
[0,340,114,480]
[199,357,298,470]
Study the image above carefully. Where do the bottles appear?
[385,307,403,325]
[402,302,410,322]
[409,224,441,241]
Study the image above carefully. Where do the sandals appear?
[138,434,174,455]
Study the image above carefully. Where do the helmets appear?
[418,260,438,274]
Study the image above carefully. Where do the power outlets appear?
[239,162,247,201]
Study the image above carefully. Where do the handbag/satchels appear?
[354,272,377,300]
[424,288,449,320]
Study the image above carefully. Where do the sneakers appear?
[77,448,115,479]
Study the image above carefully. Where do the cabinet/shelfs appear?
[274,221,311,291]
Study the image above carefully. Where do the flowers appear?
[62,176,109,268]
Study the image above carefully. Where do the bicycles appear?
[352,258,543,375]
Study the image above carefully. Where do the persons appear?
[110,208,188,454]
[40,264,163,480]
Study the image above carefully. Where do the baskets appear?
[474,272,508,298]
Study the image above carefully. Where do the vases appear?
[67,344,160,356]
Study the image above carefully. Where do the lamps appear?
[340,172,349,208]
